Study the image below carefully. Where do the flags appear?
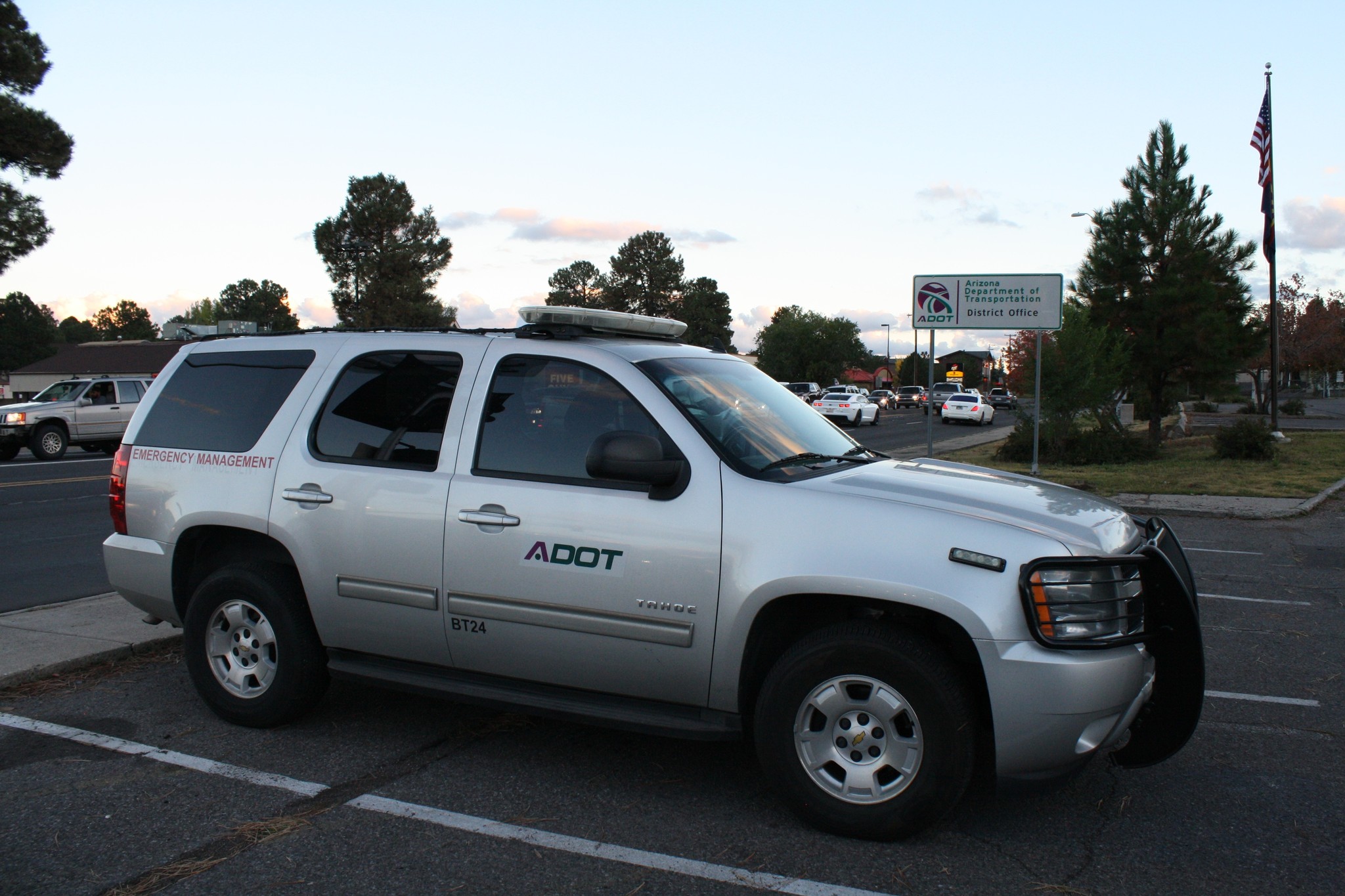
[1250,89,1276,263]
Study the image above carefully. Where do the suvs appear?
[103,325,1205,843]
[0,375,157,460]
[825,384,860,394]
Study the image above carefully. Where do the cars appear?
[987,388,1017,410]
[735,381,830,421]
[941,392,994,426]
[965,388,980,395]
[866,390,897,410]
[858,388,870,396]
[812,392,880,427]
[895,386,925,408]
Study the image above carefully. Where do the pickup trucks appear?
[920,382,966,415]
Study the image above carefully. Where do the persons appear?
[91,386,109,405]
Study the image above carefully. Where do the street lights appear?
[907,315,918,386]
[1005,335,1017,373]
[881,324,889,390]
